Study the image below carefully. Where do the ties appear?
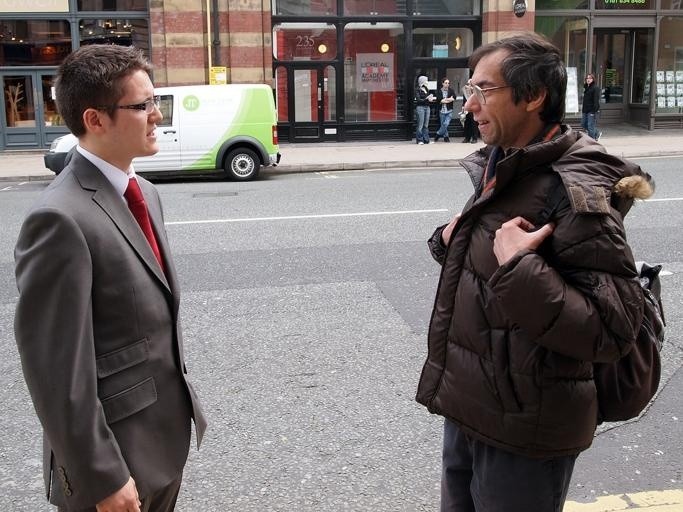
[124,178,163,268]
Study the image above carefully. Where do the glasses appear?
[461,85,513,104]
[94,96,161,114]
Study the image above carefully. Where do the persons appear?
[582,73,602,139]
[411,30,656,509]
[415,75,434,145]
[14,46,208,509]
[434,76,457,140]
[464,79,479,145]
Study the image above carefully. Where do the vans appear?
[42,83,282,183]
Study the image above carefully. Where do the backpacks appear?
[593,260,664,427]
